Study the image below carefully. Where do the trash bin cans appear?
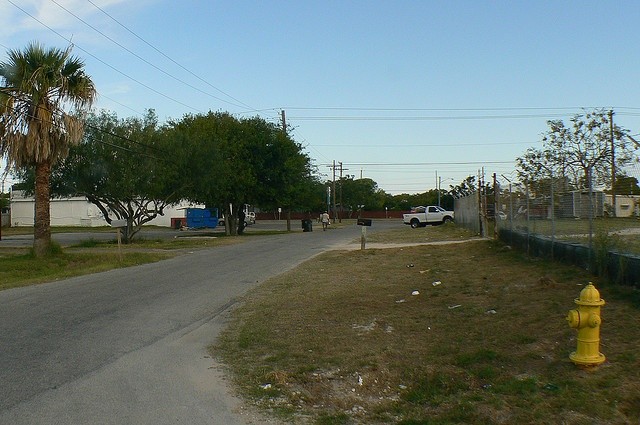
[171,207,217,228]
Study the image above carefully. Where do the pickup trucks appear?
[486,211,507,221]
[403,206,455,229]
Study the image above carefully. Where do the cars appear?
[218,215,252,226]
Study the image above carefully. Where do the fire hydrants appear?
[566,281,607,364]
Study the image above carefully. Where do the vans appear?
[249,212,256,224]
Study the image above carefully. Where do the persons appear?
[321,210,329,231]
